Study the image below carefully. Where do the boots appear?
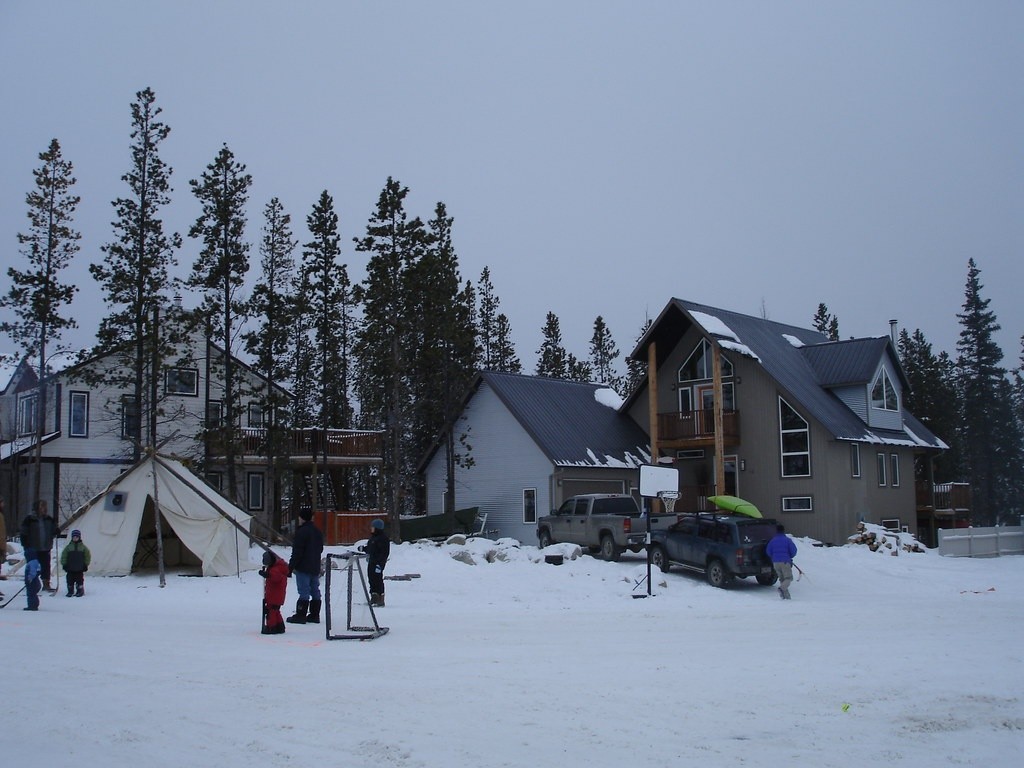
[370,593,384,606]
[75,586,84,597]
[286,600,310,624]
[306,600,321,623]
[66,586,74,597]
[42,579,56,591]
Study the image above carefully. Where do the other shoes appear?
[778,587,791,599]
[0,592,5,601]
[23,606,38,611]
[261,623,285,634]
[287,569,292,577]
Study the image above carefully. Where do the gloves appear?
[374,564,381,574]
[63,566,65,570]
[259,570,268,578]
[84,566,88,571]
[357,546,364,552]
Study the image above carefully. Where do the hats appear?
[300,508,312,522]
[776,525,785,531]
[72,530,81,537]
[371,519,384,529]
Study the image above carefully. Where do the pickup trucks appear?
[536,493,678,563]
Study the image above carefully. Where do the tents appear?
[56,450,262,577]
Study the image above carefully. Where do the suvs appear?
[649,515,784,589]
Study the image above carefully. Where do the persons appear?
[766,525,797,599]
[259,552,289,635]
[286,513,324,624]
[0,497,91,611]
[357,519,390,608]
